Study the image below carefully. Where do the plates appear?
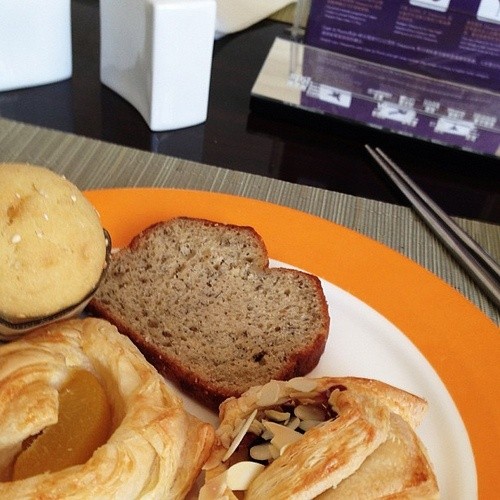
[110,248,479,500]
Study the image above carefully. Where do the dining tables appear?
[0,111,500,500]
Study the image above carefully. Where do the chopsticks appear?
[365,143,500,307]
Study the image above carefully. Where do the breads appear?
[0,317,213,500]
[196,376,441,500]
[83,217,330,413]
[0,161,114,341]
